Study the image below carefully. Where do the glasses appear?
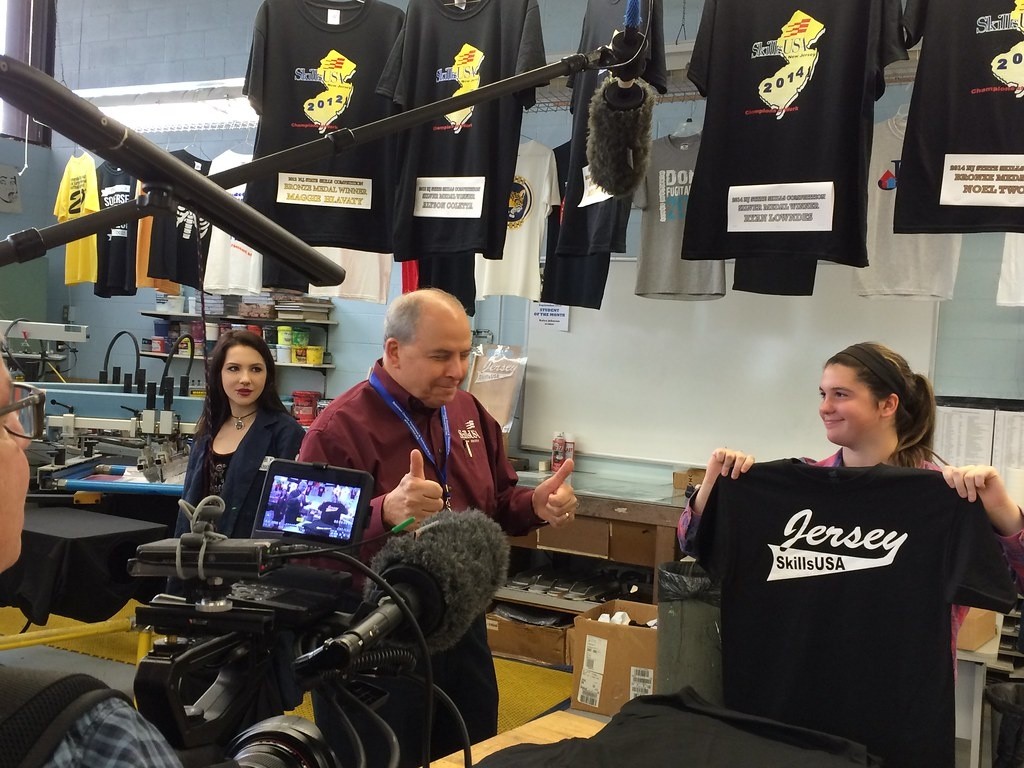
[1,383,48,441]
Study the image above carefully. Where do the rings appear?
[565,513,570,519]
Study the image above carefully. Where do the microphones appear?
[326,509,511,665]
[587,0,654,199]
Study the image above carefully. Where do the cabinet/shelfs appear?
[509,468,691,617]
[138,311,339,375]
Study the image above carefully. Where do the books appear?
[237,283,336,321]
[194,292,242,316]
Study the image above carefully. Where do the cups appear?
[140,319,332,367]
[166,295,185,313]
[291,391,321,426]
[538,461,550,470]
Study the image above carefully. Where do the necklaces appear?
[231,409,258,431]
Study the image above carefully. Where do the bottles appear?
[552,433,565,473]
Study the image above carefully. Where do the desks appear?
[419,710,607,768]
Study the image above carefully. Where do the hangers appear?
[520,134,532,141]
[184,128,212,161]
[230,126,254,150]
[72,142,85,155]
[162,131,171,151]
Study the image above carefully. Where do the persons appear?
[1,354,185,767]
[272,481,348,529]
[677,340,1024,689]
[297,287,580,768]
[166,330,306,599]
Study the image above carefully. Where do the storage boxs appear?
[485,612,575,670]
[571,599,658,716]
